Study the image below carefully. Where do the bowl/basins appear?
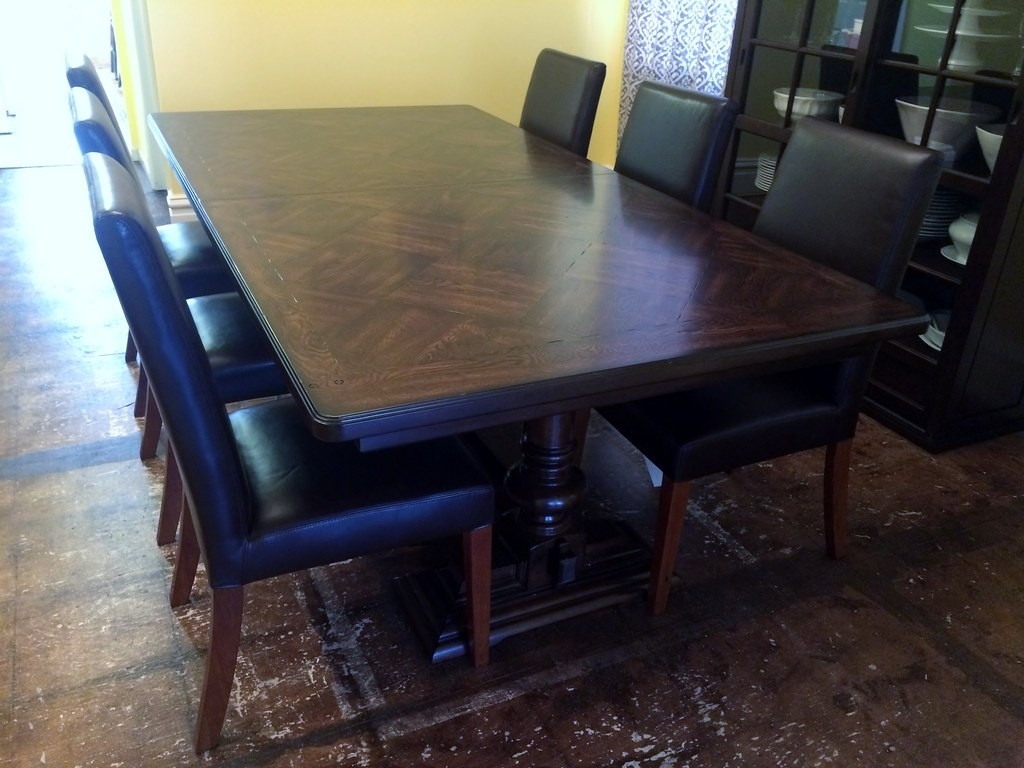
[895,97,1001,161]
[773,87,845,121]
[976,124,1006,173]
[926,313,949,347]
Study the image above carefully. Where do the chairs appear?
[66,49,938,754]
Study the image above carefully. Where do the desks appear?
[151,103,928,668]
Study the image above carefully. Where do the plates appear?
[918,334,941,352]
[940,245,966,266]
[754,151,778,191]
[916,189,964,241]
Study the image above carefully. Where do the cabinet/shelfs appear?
[720,1,1024,453]
